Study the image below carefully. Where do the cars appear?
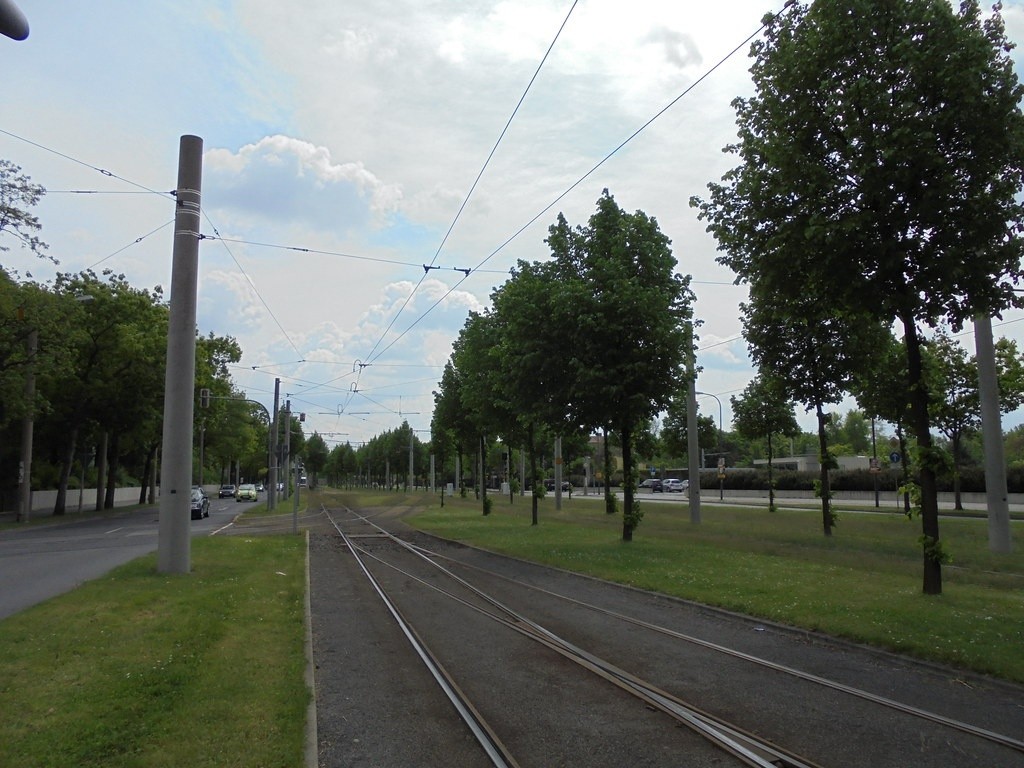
[237,484,258,502]
[219,485,238,498]
[277,482,285,490]
[256,484,265,492]
[639,479,663,492]
[681,479,689,492]
[191,484,211,520]
[662,478,683,493]
[562,482,573,492]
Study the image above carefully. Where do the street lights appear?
[695,391,724,458]
